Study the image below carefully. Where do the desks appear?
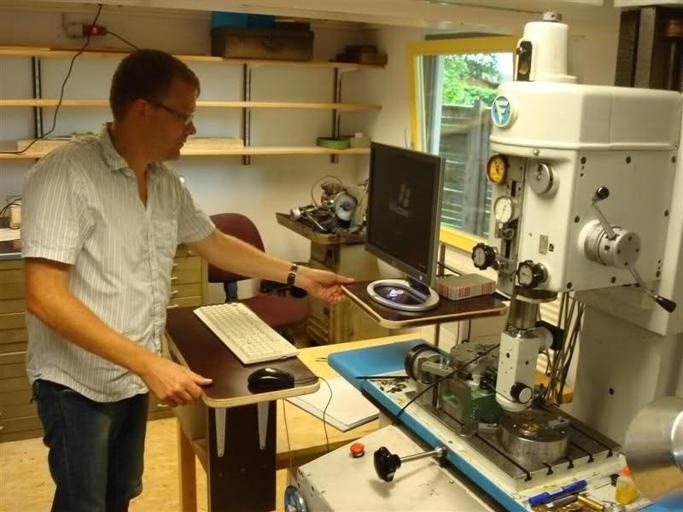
[341,274,508,348]
[165,298,320,512]
[172,334,434,512]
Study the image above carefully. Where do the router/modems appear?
[436,273,496,301]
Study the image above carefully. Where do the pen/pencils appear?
[529,467,618,509]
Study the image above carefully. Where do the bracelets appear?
[287,263,297,285]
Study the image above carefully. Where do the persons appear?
[20,49,356,512]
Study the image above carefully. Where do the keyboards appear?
[193,302,301,365]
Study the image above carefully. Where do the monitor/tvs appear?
[364,141,445,312]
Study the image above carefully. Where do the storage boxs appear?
[211,27,315,61]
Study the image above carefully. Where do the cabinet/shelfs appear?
[0,46,382,165]
[278,213,391,345]
[0,248,208,442]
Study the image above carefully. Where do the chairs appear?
[208,214,312,345]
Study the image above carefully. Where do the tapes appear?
[317,136,370,149]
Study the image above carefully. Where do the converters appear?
[68,23,107,37]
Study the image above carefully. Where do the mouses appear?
[248,366,296,390]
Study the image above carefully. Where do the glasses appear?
[150,97,194,127]
